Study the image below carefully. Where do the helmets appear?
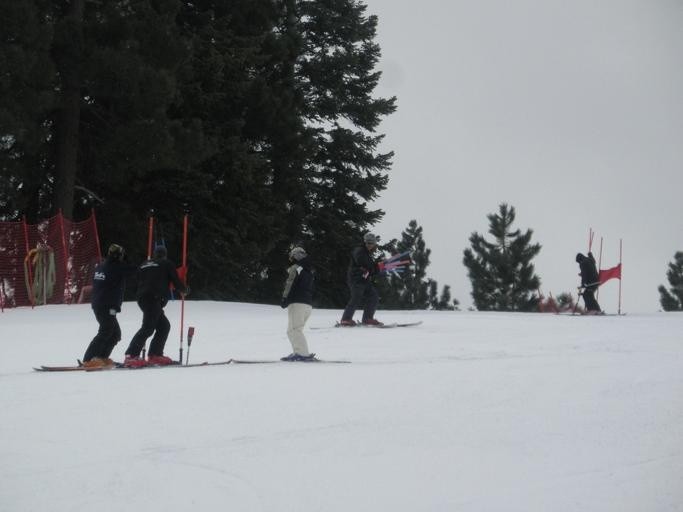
[288,246,308,264]
[362,231,377,245]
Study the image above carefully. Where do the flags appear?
[598,262,623,283]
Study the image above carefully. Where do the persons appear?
[281,247,317,361]
[82,243,138,367]
[123,245,190,367]
[575,253,602,315]
[341,233,379,326]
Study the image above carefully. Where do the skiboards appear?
[116,359,232,368]
[33,359,136,371]
[311,323,423,328]
[555,310,629,315]
[236,360,353,364]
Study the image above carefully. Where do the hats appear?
[153,244,168,260]
[108,242,125,260]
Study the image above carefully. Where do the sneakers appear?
[84,357,114,368]
[148,355,171,365]
[361,317,379,324]
[341,319,356,325]
[124,354,147,366]
